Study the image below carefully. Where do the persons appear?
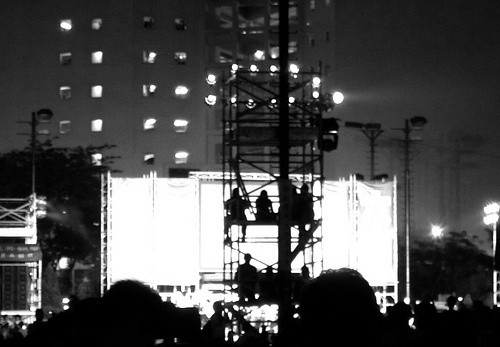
[223,185,315,248]
[0,254,500,347]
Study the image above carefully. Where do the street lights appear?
[30,107,54,194]
[483,203,500,308]
[403,115,430,303]
